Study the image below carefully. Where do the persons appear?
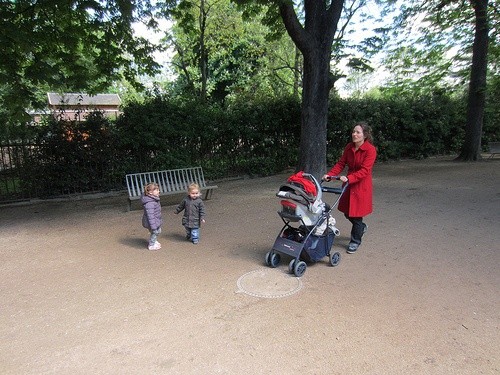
[141,182,162,250]
[322,120,377,254]
[175,183,205,244]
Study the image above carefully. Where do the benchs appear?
[125,167,219,212]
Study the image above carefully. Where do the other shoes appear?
[186,235,191,239]
[361,223,368,238]
[148,241,161,250]
[193,240,199,244]
[347,241,358,254]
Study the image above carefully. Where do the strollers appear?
[264,174,348,278]
